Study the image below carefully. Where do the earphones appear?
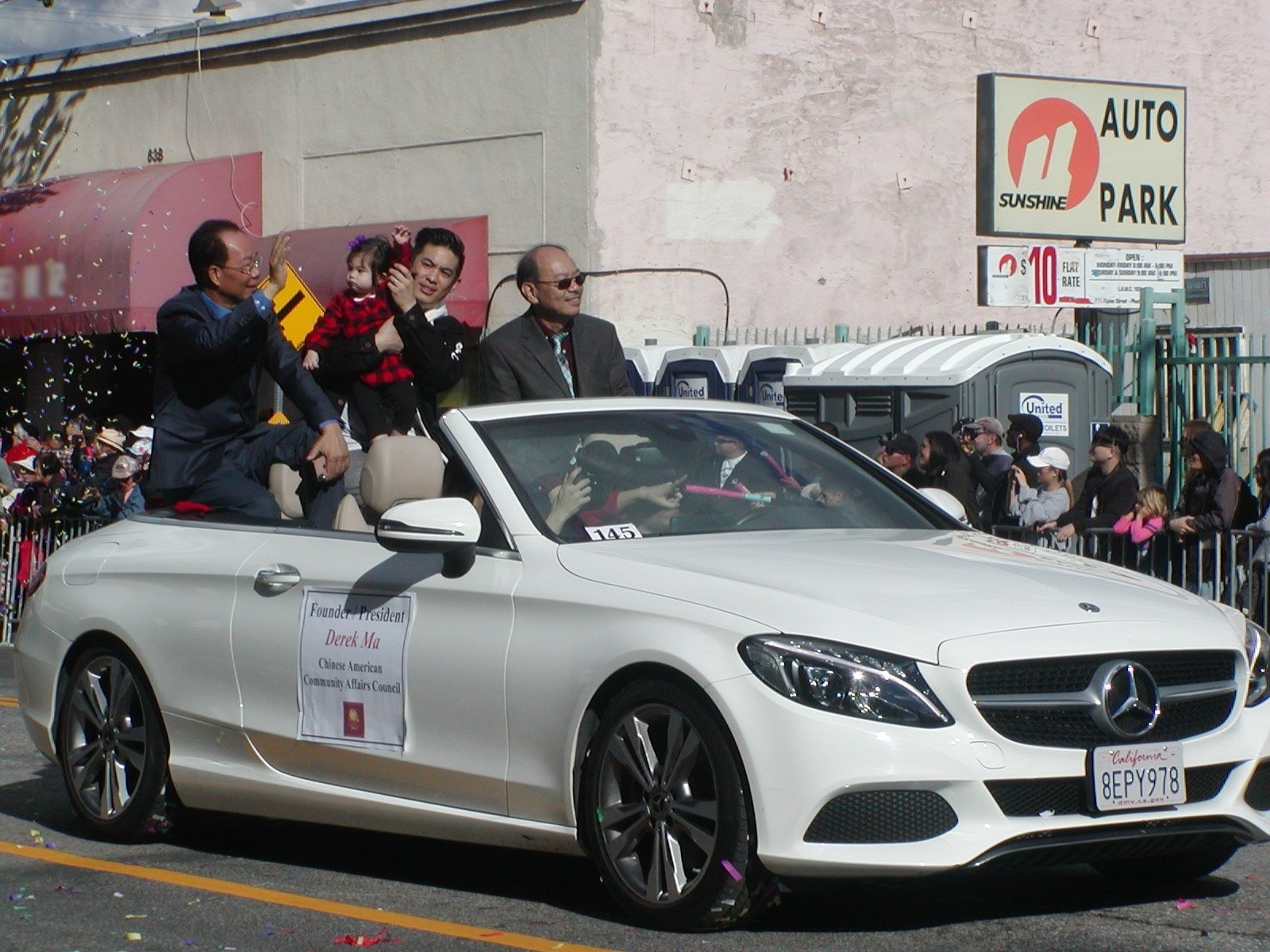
[1018,434,1023,438]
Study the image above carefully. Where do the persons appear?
[302,224,413,449]
[965,418,1011,534]
[697,434,854,515]
[1005,447,1073,553]
[479,244,688,537]
[1235,449,1270,631]
[1113,482,1170,579]
[1169,430,1252,601]
[879,433,924,488]
[0,414,154,632]
[959,413,1043,541]
[314,227,475,452]
[145,218,349,526]
[919,431,979,528]
[1037,426,1140,567]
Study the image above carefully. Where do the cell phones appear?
[310,454,327,482]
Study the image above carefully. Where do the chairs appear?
[336,432,445,530]
[270,463,305,518]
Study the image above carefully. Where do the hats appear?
[112,456,138,478]
[1189,431,1226,475]
[965,416,1004,444]
[127,425,155,440]
[95,430,127,454]
[879,432,919,458]
[1027,446,1070,471]
[12,455,36,471]
[125,437,153,456]
[1008,412,1043,441]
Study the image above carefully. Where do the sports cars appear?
[17,398,1270,933]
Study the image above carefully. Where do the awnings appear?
[254,215,488,326]
[0,153,262,341]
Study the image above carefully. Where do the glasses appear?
[533,272,586,290]
[216,257,263,275]
[1253,465,1266,476]
[1092,442,1115,450]
[717,438,736,445]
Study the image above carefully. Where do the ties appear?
[550,333,575,398]
